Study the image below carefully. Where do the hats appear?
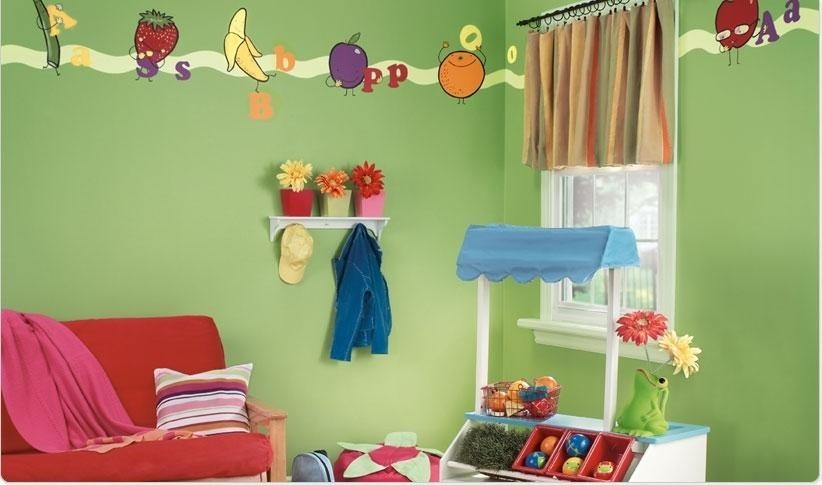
[278,223,314,284]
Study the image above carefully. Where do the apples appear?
[328,42,367,88]
[714,0,759,49]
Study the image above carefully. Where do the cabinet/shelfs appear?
[438,225,711,482]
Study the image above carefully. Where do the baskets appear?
[480,381,561,420]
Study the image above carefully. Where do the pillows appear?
[150,361,256,439]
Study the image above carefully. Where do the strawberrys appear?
[134,9,179,64]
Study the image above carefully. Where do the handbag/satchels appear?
[292,451,334,482]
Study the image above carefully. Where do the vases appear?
[278,187,385,218]
[610,366,671,439]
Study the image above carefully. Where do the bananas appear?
[223,7,269,83]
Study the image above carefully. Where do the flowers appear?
[275,157,388,200]
[616,306,701,380]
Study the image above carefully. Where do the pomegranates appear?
[489,377,557,411]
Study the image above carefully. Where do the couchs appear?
[0,314,286,482]
